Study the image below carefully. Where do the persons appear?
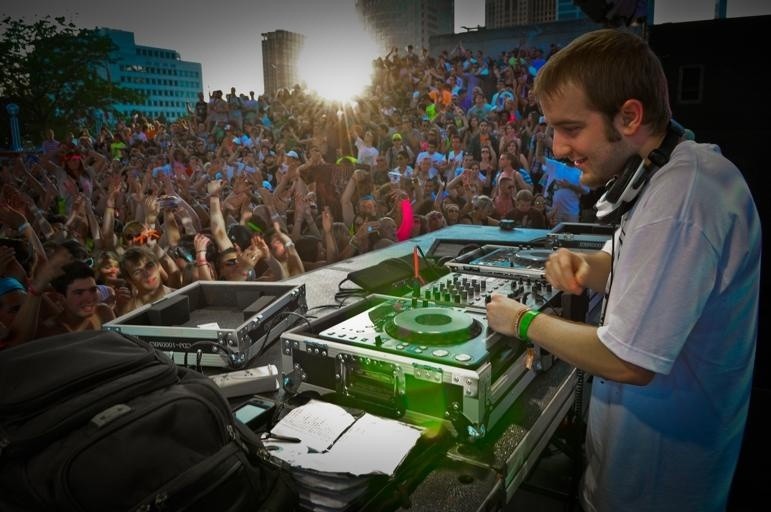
[483,29,762,511]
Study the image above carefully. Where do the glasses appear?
[126,231,141,241]
[225,258,240,266]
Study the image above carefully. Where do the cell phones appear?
[232,397,278,431]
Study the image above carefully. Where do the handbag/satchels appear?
[0,326,296,512]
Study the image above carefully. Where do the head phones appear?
[592,117,687,233]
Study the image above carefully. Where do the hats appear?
[2,277,25,294]
[232,138,240,144]
[224,125,232,131]
[286,151,298,160]
[393,134,402,140]
[63,154,81,161]
